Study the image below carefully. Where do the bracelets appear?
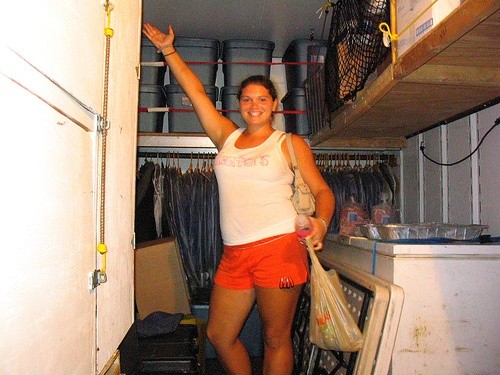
[164,49,177,57]
[318,217,328,235]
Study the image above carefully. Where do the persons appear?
[141,22,337,375]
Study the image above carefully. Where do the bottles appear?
[294,214,313,239]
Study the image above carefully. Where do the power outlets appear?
[87,269,101,290]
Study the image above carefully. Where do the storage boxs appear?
[134,236,264,375]
[137,36,329,133]
[395,0,465,59]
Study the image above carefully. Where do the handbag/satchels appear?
[306,236,364,352]
[284,132,316,215]
[136,311,197,339]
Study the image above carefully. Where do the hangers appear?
[136,148,401,188]
